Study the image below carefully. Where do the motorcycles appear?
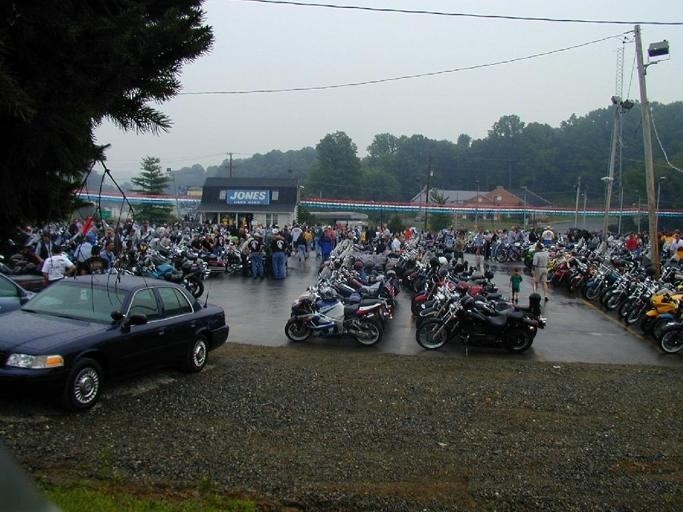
[127,231,251,298]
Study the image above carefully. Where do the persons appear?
[1,217,309,291]
[307,222,435,258]
[437,222,557,300]
[559,225,683,269]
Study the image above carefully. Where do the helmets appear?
[457,281,468,295]
[354,261,363,270]
[461,296,474,310]
[321,288,337,301]
[439,257,447,266]
[471,285,483,296]
[349,293,361,304]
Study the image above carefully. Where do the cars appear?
[0,274,229,411]
[0,271,36,314]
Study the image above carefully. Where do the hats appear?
[93,245,102,254]
